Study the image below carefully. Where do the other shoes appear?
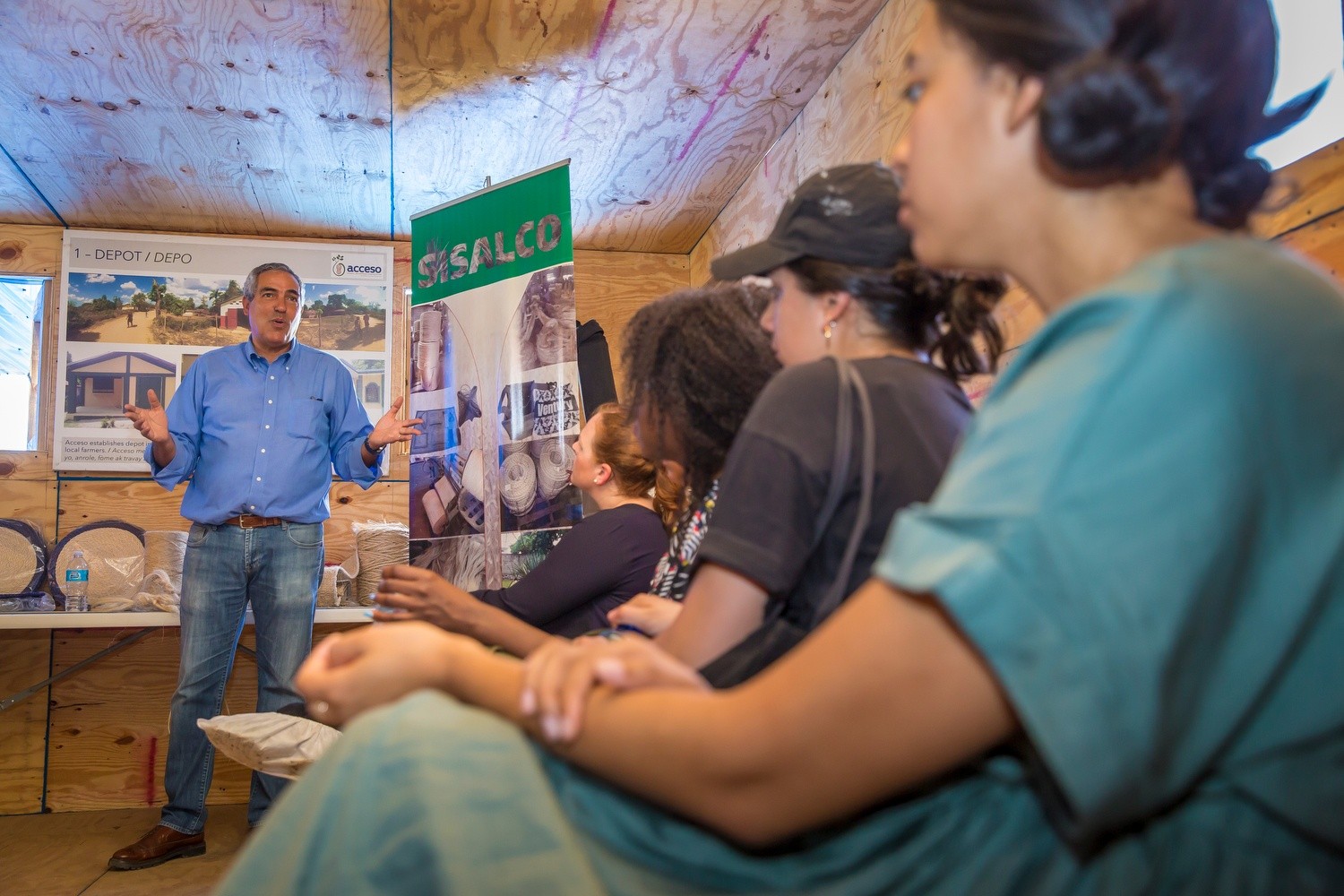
[197,702,347,780]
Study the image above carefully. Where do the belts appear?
[226,514,289,529]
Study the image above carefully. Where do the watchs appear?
[364,432,387,454]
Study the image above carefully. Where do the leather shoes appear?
[108,825,206,870]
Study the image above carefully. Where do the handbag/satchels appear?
[698,354,872,686]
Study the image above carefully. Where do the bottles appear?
[65,552,90,613]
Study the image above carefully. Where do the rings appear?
[398,434,401,441]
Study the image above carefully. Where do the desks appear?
[1,605,396,711]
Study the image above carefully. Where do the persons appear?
[108,263,422,871]
[220,0,1344,896]
[126,310,134,328]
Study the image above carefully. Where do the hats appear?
[711,162,910,280]
